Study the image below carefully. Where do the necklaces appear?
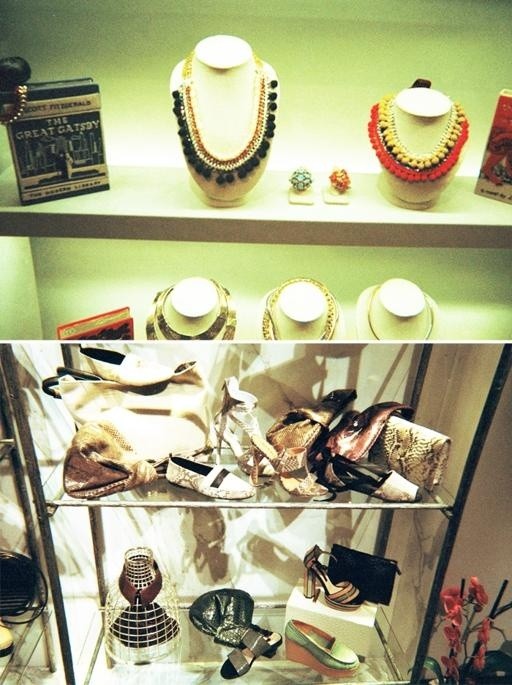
[365,88,470,184]
[367,282,435,341]
[118,555,164,608]
[0,83,29,126]
[171,48,277,186]
[260,275,341,341]
[142,277,238,341]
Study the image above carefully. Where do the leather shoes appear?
[220,626,282,680]
[323,450,424,503]
[165,451,257,501]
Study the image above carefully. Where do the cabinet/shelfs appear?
[0,344,512,685]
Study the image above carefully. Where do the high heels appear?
[302,544,364,613]
[213,374,278,480]
[249,433,330,498]
[283,619,360,678]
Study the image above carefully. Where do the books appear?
[55,305,136,340]
[4,78,111,207]
[474,88,512,208]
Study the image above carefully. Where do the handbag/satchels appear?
[367,413,452,492]
[326,543,402,607]
[41,363,222,481]
